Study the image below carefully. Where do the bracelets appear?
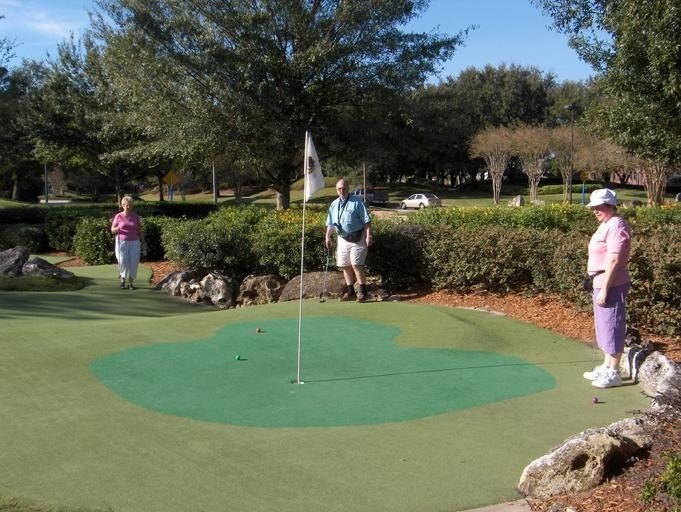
[368,235,374,238]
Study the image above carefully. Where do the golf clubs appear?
[319,241,330,303]
[118,232,121,279]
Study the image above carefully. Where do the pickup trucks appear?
[348,187,389,205]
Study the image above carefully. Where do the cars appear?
[400,193,441,211]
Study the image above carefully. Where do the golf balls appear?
[256,328,261,333]
[236,355,240,360]
[592,397,598,403]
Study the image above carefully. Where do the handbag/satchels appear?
[337,227,362,243]
[584,275,595,292]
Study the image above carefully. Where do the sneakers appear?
[128,284,135,290]
[591,367,623,388]
[120,281,125,288]
[584,363,611,380]
[338,292,357,301]
[355,291,366,303]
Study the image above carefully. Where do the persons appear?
[325,179,374,303]
[582,187,633,389]
[110,196,147,290]
[134,183,146,194]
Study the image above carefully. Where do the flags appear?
[303,132,327,205]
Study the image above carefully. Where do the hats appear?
[585,188,617,208]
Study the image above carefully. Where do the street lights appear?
[563,104,576,204]
